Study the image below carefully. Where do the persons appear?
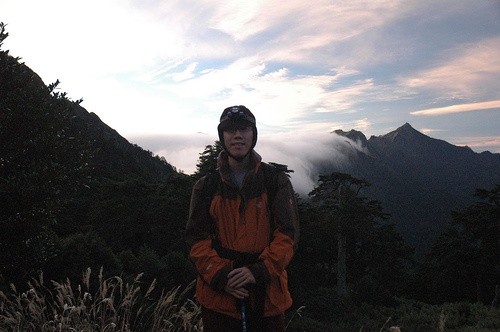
[183,106,300,332]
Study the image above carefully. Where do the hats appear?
[219,105,256,127]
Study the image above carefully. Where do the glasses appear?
[221,125,253,133]
